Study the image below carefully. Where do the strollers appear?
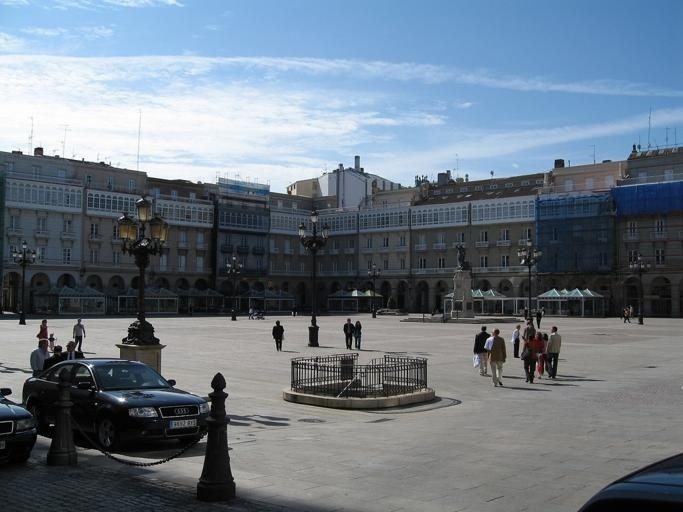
[254,310,264,320]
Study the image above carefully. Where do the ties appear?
[69,352,72,360]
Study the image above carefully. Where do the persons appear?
[473,310,561,387]
[353,321,361,349]
[272,321,284,351]
[29,318,85,377]
[343,319,354,350]
[291,307,297,320]
[622,307,631,323]
[247,306,254,319]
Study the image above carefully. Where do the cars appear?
[23,357,210,451]
[577,450,681,510]
[0,385,38,464]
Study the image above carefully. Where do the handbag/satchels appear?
[473,354,480,368]
[521,349,531,360]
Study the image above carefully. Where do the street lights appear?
[517,238,544,324]
[367,262,381,319]
[295,209,330,347]
[224,254,243,321]
[11,240,36,325]
[628,253,652,325]
[118,195,170,347]
